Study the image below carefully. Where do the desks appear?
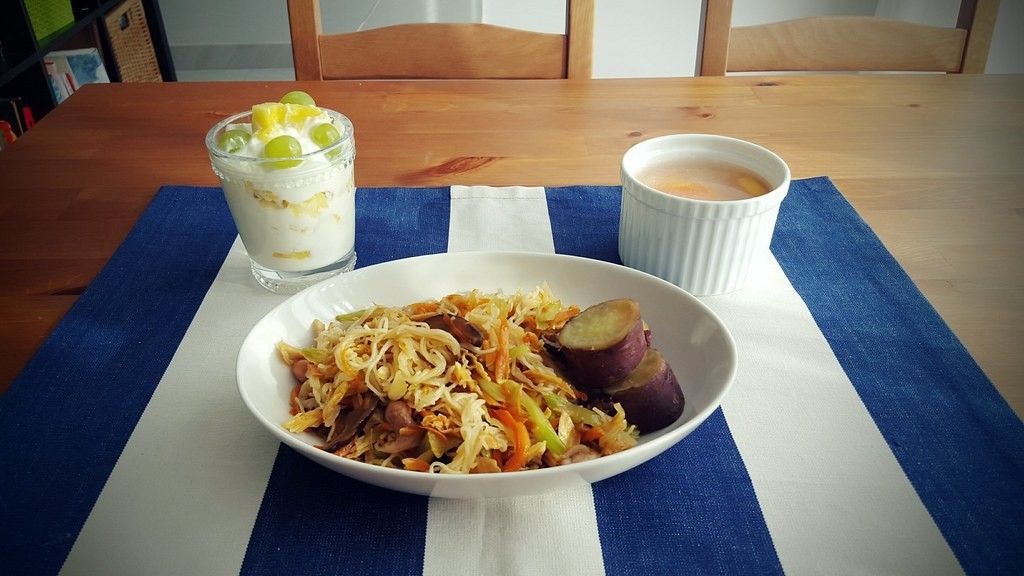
[0,73,1024,428]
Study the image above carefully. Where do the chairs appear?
[285,1,595,81]
[701,0,1001,74]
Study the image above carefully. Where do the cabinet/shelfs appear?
[0,0,177,138]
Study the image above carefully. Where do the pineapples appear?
[250,103,324,143]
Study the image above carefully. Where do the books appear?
[0,95,36,138]
[43,46,111,105]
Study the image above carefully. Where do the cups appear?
[617,134,792,298]
[203,106,360,292]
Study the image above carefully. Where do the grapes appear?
[219,130,251,152]
[265,135,303,169]
[313,123,342,157]
[281,91,316,107]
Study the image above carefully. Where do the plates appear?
[236,249,739,499]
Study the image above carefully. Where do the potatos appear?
[550,299,685,432]
